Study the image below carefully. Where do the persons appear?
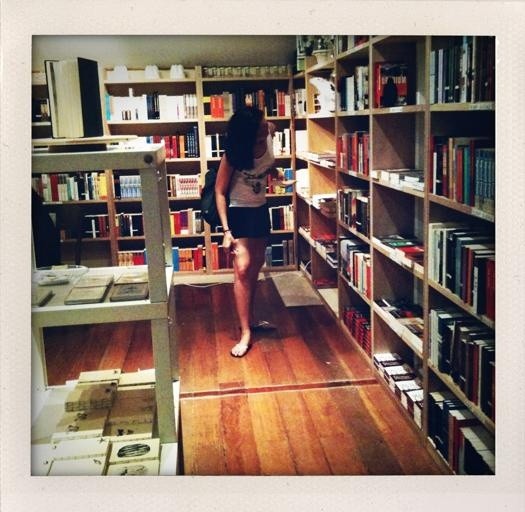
[216,107,276,356]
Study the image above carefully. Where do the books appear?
[295,129,308,159]
[109,283,148,301]
[43,440,110,475]
[265,165,293,194]
[82,213,110,233]
[166,173,201,197]
[201,65,288,78]
[114,171,142,199]
[428,37,494,105]
[31,170,108,201]
[106,439,163,474]
[338,239,371,301]
[428,129,495,216]
[342,305,371,358]
[168,207,204,236]
[337,129,369,177]
[374,233,425,267]
[427,308,495,424]
[336,187,371,238]
[299,258,312,279]
[294,168,309,199]
[211,240,294,270]
[31,286,53,308]
[114,273,148,283]
[374,298,424,343]
[110,126,199,158]
[102,388,156,438]
[309,150,335,168]
[64,379,122,412]
[43,56,102,138]
[64,286,108,305]
[207,128,291,158]
[115,214,144,235]
[338,66,368,112]
[372,168,424,192]
[427,220,495,321]
[105,87,198,121]
[211,87,290,117]
[311,193,336,216]
[374,40,426,108]
[293,87,307,116]
[170,244,206,272]
[73,274,114,287]
[267,203,292,233]
[311,231,338,268]
[298,223,310,240]
[116,248,148,267]
[373,352,423,430]
[51,410,110,441]
[428,391,495,475]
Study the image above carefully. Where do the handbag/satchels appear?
[199,167,230,228]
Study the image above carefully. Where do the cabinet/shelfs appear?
[292,35,495,475]
[102,66,300,272]
[31,139,185,476]
[32,71,113,242]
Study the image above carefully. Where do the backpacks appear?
[201,165,227,227]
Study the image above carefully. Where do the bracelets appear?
[222,230,230,232]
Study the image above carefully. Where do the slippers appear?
[239,320,278,332]
[230,339,252,358]
